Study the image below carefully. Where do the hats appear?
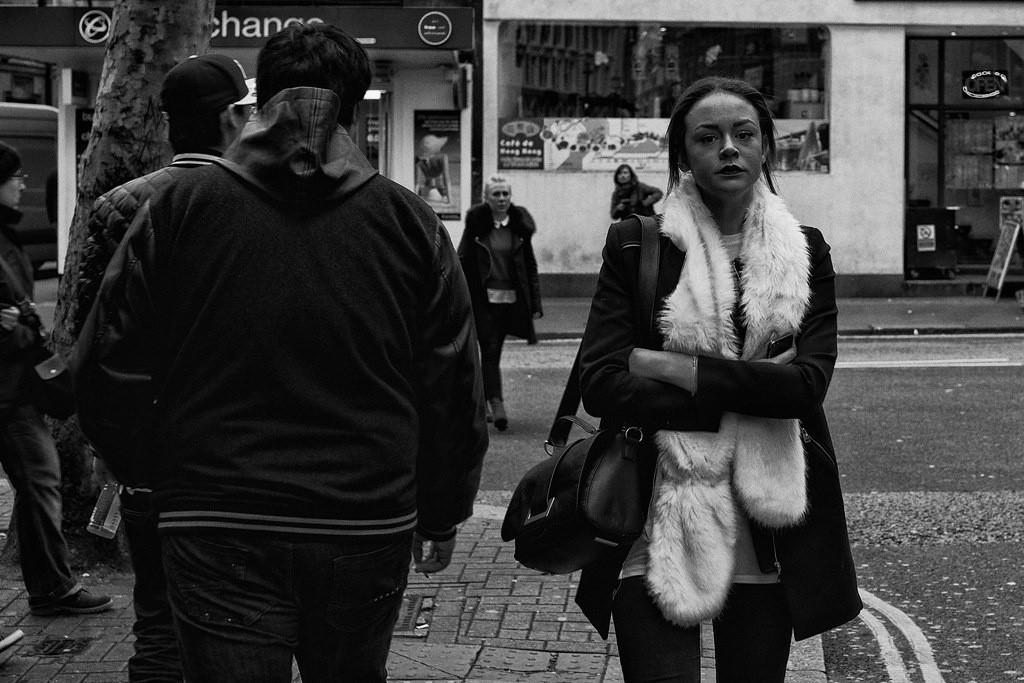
[1,143,21,185]
[419,135,448,158]
[160,52,258,113]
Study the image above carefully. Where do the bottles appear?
[87,475,122,540]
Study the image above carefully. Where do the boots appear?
[489,396,509,432]
[485,404,492,421]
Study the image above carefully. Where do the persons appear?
[610,164,664,221]
[415,135,453,204]
[68,21,490,683]
[457,175,545,431]
[575,78,863,683]
[0,142,113,617]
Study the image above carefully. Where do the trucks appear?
[1,103,60,271]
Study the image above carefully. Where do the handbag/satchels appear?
[32,349,75,420]
[501,416,644,574]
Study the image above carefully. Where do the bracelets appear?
[692,355,697,397]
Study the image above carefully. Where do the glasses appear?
[10,174,30,183]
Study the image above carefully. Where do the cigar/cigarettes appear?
[417,561,429,578]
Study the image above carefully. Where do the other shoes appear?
[30,588,113,615]
[0,627,24,652]
